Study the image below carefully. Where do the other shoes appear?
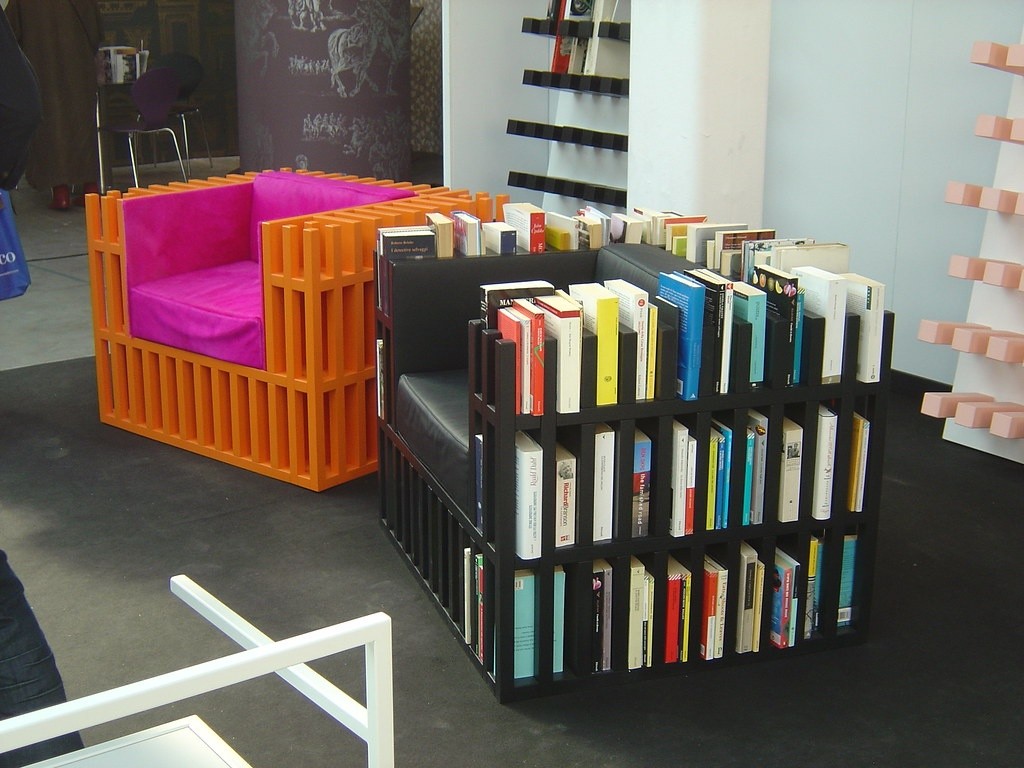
[47,185,71,209]
[78,184,101,206]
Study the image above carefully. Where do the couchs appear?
[373,240,897,708]
[84,166,509,496]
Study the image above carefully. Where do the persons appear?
[0,7,86,768]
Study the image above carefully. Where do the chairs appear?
[95,45,214,193]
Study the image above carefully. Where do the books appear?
[97,46,149,83]
[475,402,870,560]
[465,535,858,680]
[545,0,631,80]
[379,202,707,317]
[479,222,886,417]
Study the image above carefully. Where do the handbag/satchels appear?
[1,189,32,299]
